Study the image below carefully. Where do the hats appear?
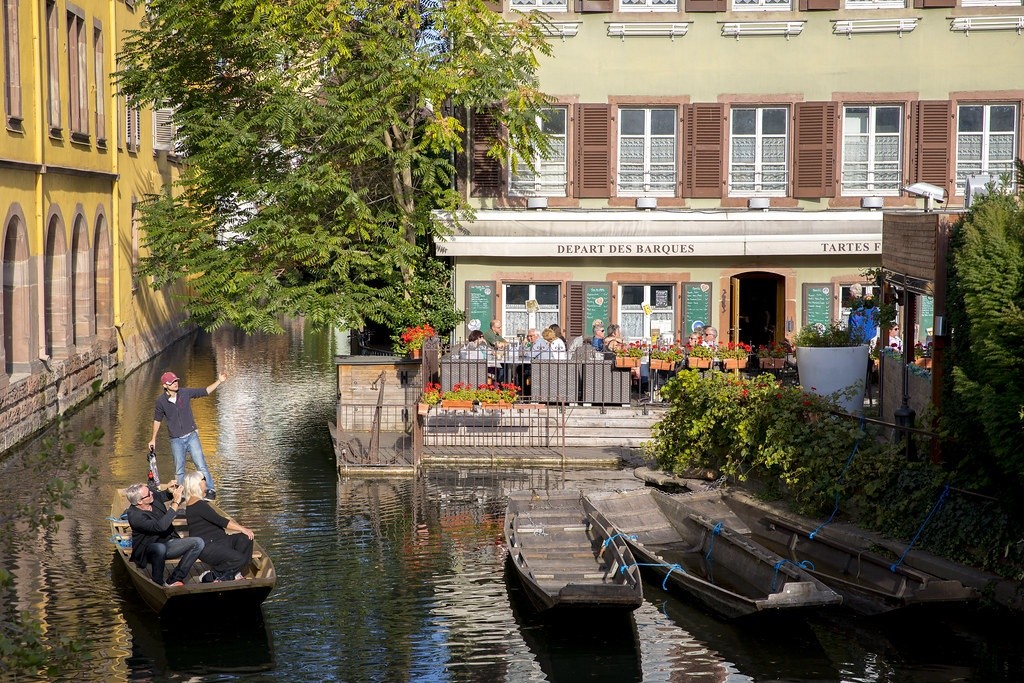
[161,372,180,386]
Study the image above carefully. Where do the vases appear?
[440,400,473,408]
[688,357,714,369]
[650,359,676,371]
[914,358,932,368]
[722,357,749,369]
[615,356,641,367]
[864,300,875,309]
[411,349,423,359]
[481,399,512,409]
[418,404,429,415]
[759,358,787,368]
[851,301,862,309]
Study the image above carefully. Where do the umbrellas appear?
[146,446,161,490]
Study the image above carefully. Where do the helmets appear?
[592,319,603,326]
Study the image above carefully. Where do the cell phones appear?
[175,484,179,489]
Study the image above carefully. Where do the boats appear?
[582,486,850,624]
[671,489,981,619]
[506,488,643,622]
[110,485,277,626]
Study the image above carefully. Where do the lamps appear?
[636,198,658,212]
[902,183,949,211]
[964,172,1000,207]
[861,196,884,211]
[527,199,548,212]
[748,197,770,212]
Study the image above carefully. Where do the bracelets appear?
[171,501,179,506]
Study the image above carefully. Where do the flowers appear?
[650,339,685,363]
[864,295,875,300]
[685,336,716,361]
[615,341,647,359]
[850,295,863,301]
[914,341,933,356]
[717,341,752,360]
[890,343,901,353]
[401,323,436,351]
[756,339,793,359]
[418,382,522,405]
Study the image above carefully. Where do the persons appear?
[868,321,903,355]
[847,283,880,345]
[468,320,718,398]
[125,479,205,587]
[182,471,254,583]
[148,370,228,502]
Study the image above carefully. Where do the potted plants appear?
[792,319,870,421]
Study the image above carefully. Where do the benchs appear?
[165,550,263,564]
[117,532,189,541]
[112,519,188,526]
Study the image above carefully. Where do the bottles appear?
[148,471,156,493]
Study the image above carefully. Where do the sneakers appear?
[204,489,216,501]
[168,496,185,509]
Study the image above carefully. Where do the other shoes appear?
[165,579,183,588]
[199,570,218,583]
[149,577,165,588]
[234,572,242,581]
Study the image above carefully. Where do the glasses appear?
[141,491,150,500]
[201,476,206,481]
[480,335,483,337]
[527,335,534,337]
[706,334,713,336]
[689,337,695,340]
[892,328,899,331]
[597,330,604,332]
